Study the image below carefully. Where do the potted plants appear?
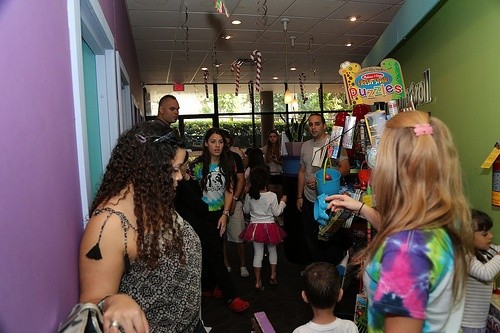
[280,114,309,156]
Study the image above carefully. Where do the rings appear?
[109,320,120,329]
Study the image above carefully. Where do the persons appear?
[326,110,474,333]
[293,262,359,333]
[79,123,209,333]
[297,114,350,262]
[155,95,180,129]
[175,127,282,276]
[461,208,500,333]
[239,165,286,288]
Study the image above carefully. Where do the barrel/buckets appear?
[315,158,342,196]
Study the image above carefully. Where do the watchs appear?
[223,211,230,216]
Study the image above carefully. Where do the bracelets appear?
[297,195,302,198]
[358,203,365,215]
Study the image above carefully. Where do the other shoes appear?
[270,275,276,281]
[255,281,263,291]
[202,286,224,298]
[296,272,305,280]
[227,267,231,272]
[240,267,250,276]
[227,297,250,312]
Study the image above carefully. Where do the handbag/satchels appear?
[57,302,105,333]
